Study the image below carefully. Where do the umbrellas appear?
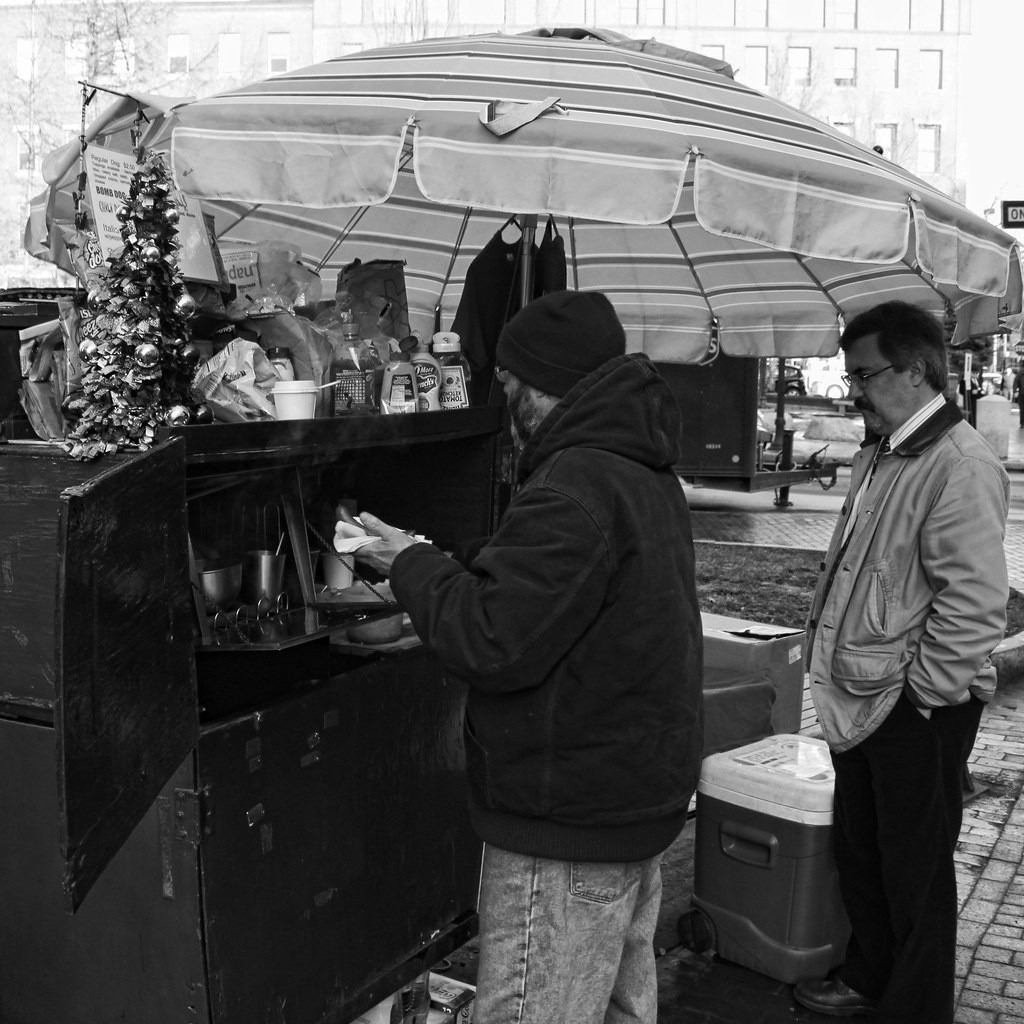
[21,23,1023,366]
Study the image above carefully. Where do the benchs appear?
[833,400,854,413]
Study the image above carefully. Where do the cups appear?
[321,552,355,588]
[269,380,319,418]
[244,551,285,605]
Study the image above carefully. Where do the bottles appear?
[381,352,418,414]
[408,344,441,412]
[332,323,374,418]
[432,331,471,409]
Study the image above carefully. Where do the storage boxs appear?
[429,972,476,1024]
[352,993,457,1024]
[700,611,807,736]
[678,735,842,984]
[703,667,776,759]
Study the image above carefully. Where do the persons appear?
[791,300,1010,1024]
[346,288,704,1024]
[1003,367,1016,400]
[960,364,987,429]
[1012,362,1024,427]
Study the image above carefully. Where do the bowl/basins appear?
[197,558,243,608]
[345,612,404,644]
